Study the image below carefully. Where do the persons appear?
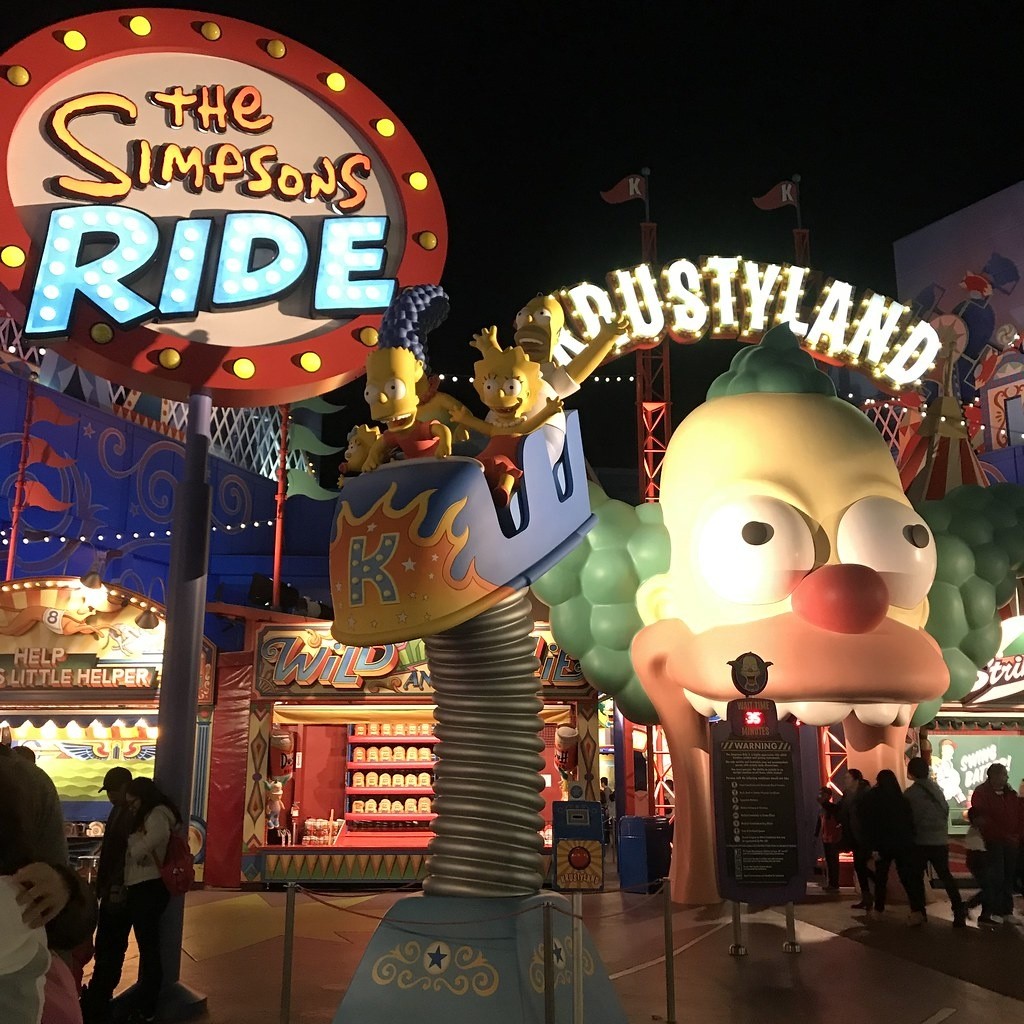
[554,726,579,790]
[961,764,1024,926]
[0,744,186,1024]
[601,777,612,843]
[266,781,286,829]
[338,294,630,510]
[813,757,966,928]
[600,790,607,845]
[609,783,616,847]
[664,780,674,843]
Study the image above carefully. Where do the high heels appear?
[851,893,873,910]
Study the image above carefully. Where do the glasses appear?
[128,798,140,809]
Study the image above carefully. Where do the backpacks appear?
[144,810,195,898]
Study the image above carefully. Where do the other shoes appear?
[872,909,885,922]
[905,910,921,926]
[79,984,110,1011]
[978,916,1000,927]
[1003,915,1024,925]
[962,902,971,919]
[822,884,839,890]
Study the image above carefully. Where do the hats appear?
[98,767,132,793]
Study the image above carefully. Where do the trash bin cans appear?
[617,815,672,895]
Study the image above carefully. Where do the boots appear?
[951,905,971,927]
